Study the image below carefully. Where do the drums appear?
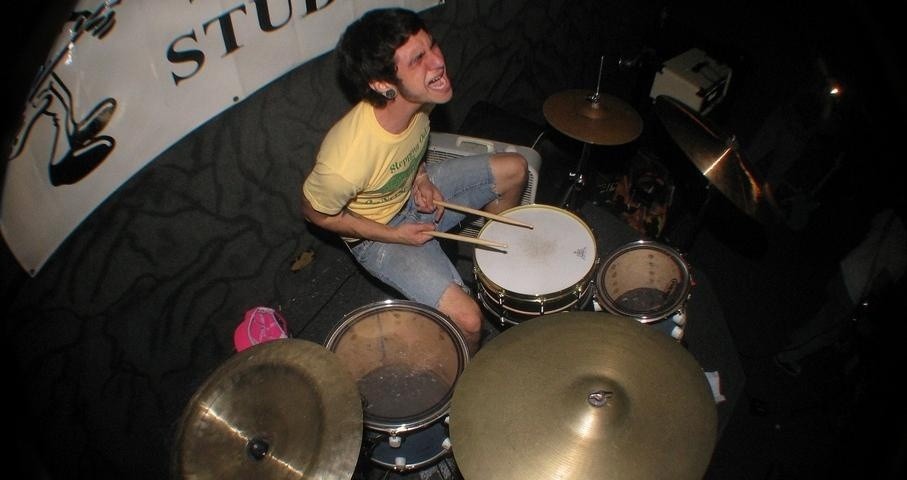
[594,237,694,344]
[322,297,469,474]
[469,204,598,329]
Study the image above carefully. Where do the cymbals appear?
[653,90,775,221]
[166,339,364,479]
[539,84,643,146]
[447,312,717,479]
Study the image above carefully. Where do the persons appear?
[302,8,528,359]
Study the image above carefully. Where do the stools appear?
[436,230,458,267]
